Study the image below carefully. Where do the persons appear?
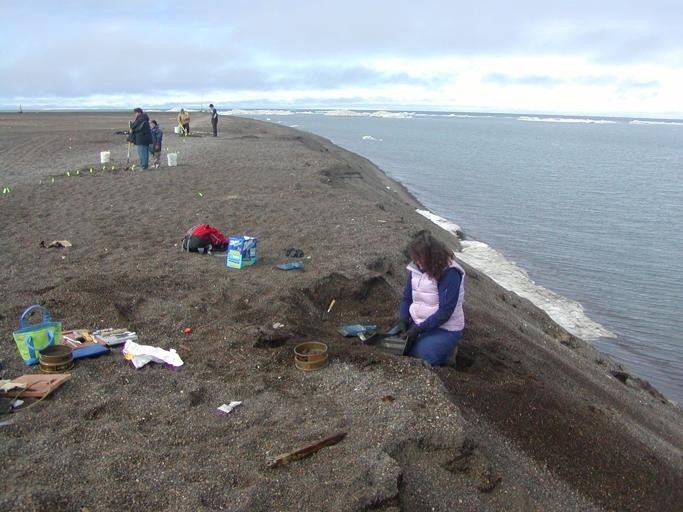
[208,104,217,137]
[176,108,190,134]
[127,108,151,171]
[145,120,162,169]
[385,228,467,368]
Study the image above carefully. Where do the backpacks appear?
[181,223,229,254]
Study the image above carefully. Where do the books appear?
[92,327,138,346]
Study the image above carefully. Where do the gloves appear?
[394,319,421,342]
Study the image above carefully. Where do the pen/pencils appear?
[63,336,81,347]
[90,335,98,343]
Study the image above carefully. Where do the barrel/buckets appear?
[100,151,111,163]
[167,153,178,166]
[174,126,180,135]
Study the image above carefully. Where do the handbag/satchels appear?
[223,234,257,269]
[10,304,62,367]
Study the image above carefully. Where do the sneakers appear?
[441,346,458,368]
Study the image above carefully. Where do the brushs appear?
[73,331,93,343]
